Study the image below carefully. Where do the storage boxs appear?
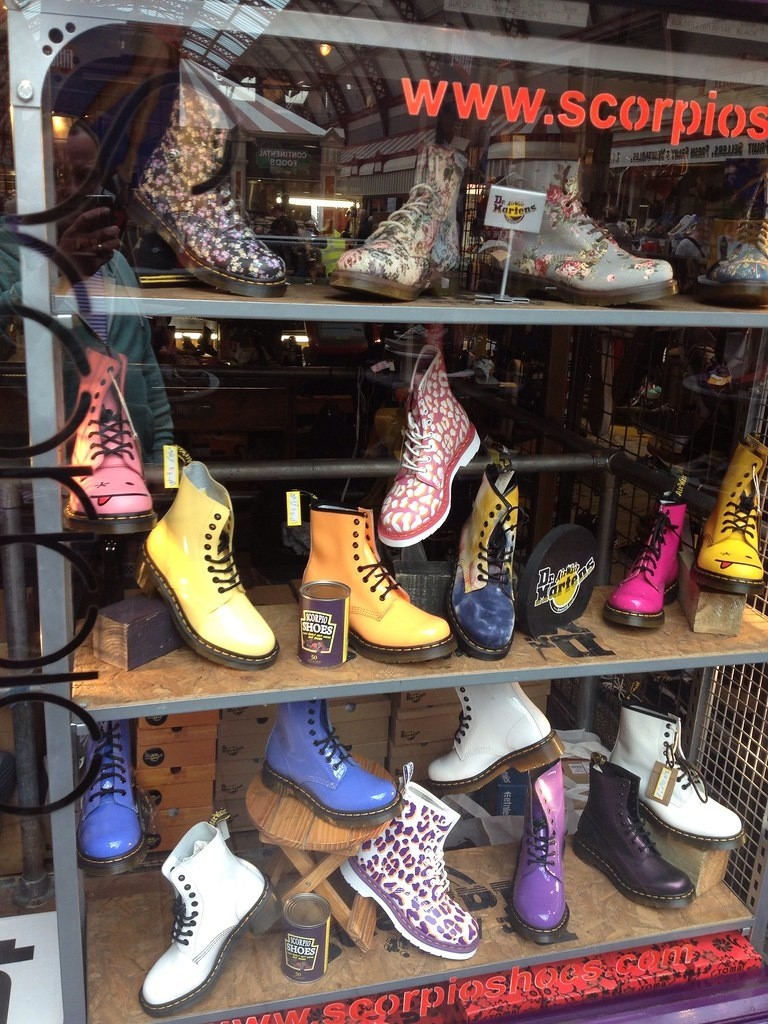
[0,586,614,877]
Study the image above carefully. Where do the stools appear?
[245,751,403,953]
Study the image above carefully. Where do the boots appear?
[605,476,687,629]
[63,348,159,534]
[341,763,479,960]
[571,754,696,905]
[139,443,280,667]
[126,88,289,298]
[511,757,571,945]
[138,820,283,1017]
[446,449,520,658]
[611,705,746,849]
[426,679,566,789]
[328,147,471,298]
[378,346,481,549]
[263,696,406,828]
[290,489,459,664]
[690,436,768,594]
[496,161,680,305]
[77,717,148,876]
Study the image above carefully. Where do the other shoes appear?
[697,217,767,305]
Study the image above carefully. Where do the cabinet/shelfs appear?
[4,0,768,1024]
